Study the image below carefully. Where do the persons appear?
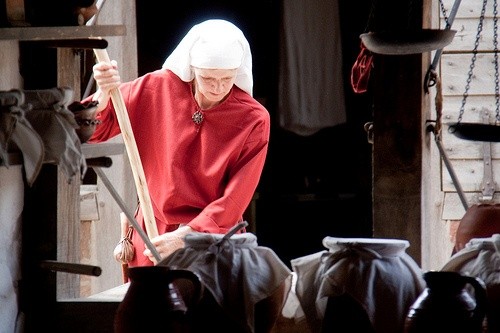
[78,20,270,284]
